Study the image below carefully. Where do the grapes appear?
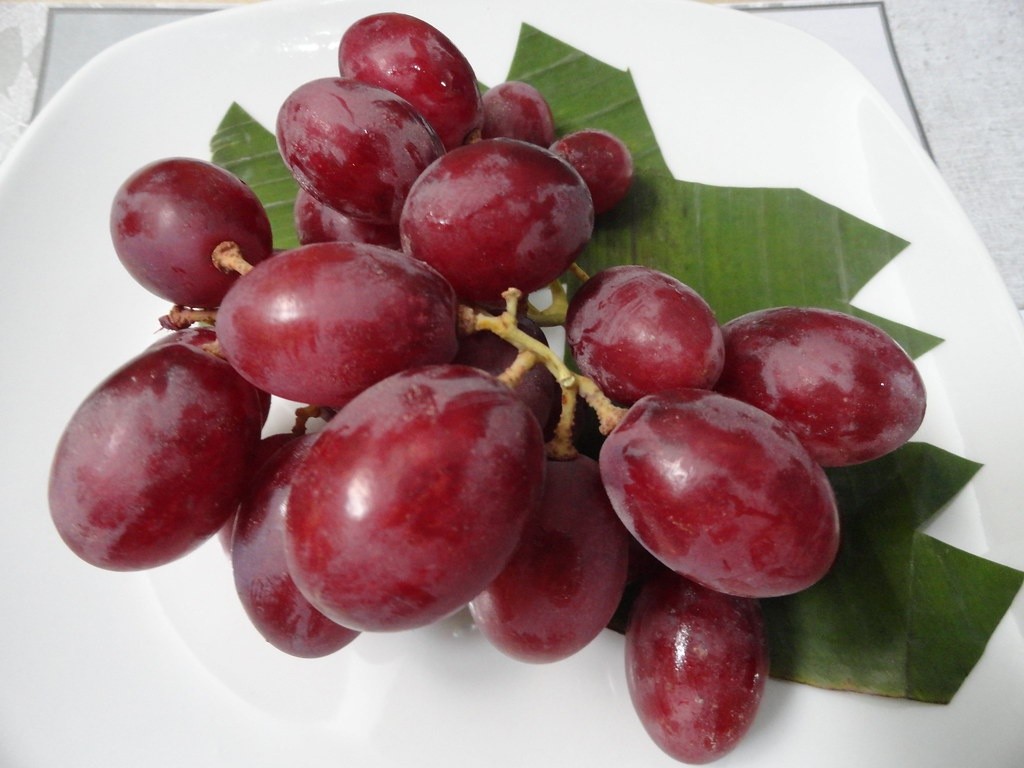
[49,12,928,767]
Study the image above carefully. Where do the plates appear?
[0,0,1024,767]
